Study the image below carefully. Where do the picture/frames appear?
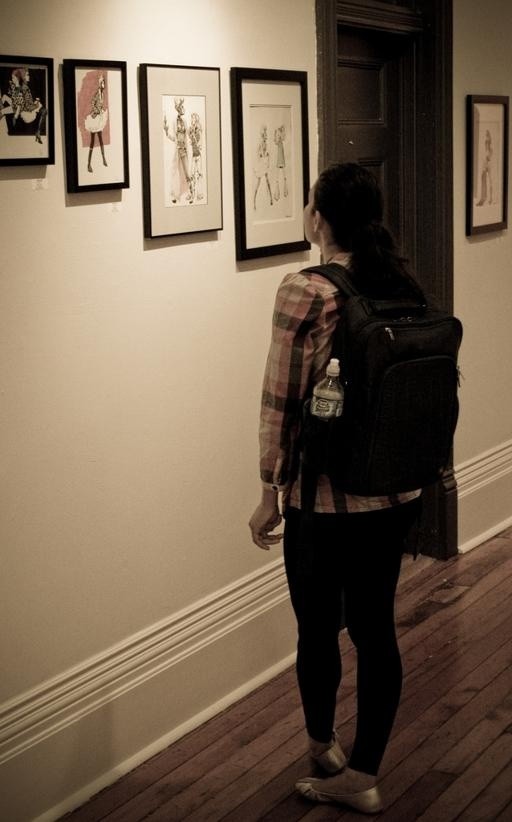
[465,94,508,236]
[0,55,55,168]
[138,62,223,239]
[61,58,129,194]
[229,66,312,262]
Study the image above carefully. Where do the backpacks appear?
[306,262,462,493]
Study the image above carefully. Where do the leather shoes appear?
[294,774,384,812]
[307,728,346,773]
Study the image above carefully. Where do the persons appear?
[1,68,23,124]
[186,110,201,205]
[86,74,108,172]
[271,126,291,201]
[244,161,419,814]
[22,66,47,145]
[477,130,494,210]
[253,126,274,212]
[163,99,193,204]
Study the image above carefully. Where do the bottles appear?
[309,358,347,419]
